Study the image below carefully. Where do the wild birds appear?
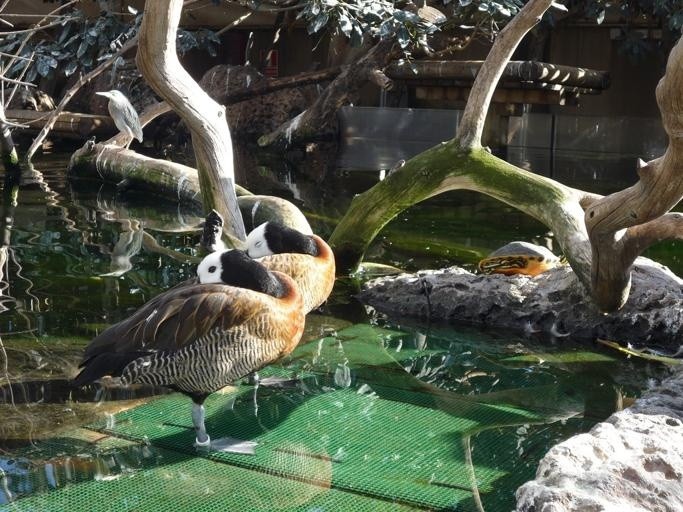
[93,90,144,152]
[67,248,307,455]
[237,221,337,390]
[98,220,145,278]
